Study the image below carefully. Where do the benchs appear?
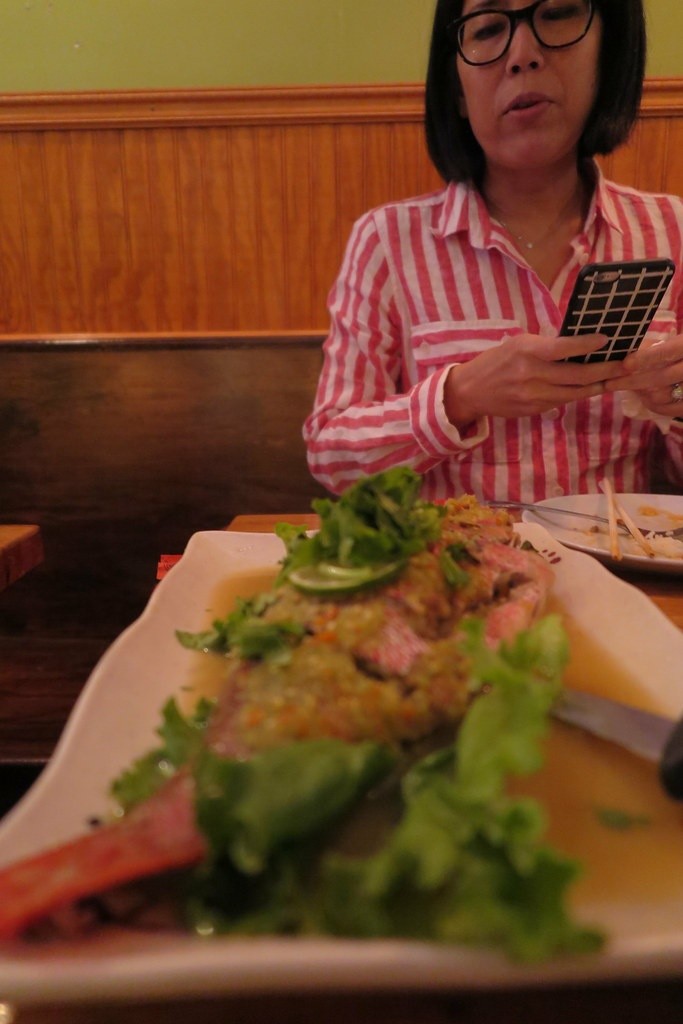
[0,332,332,817]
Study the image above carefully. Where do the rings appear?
[547,408,559,420]
[671,384,683,403]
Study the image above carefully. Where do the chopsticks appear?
[600,476,661,565]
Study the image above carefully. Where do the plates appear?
[0,533,683,1024]
[522,494,683,566]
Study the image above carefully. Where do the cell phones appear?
[555,257,676,364]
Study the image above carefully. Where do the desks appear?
[227,514,683,639]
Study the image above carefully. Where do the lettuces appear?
[112,464,611,966]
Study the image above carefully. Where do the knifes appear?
[548,683,683,798]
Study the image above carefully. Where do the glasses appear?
[446,0,596,66]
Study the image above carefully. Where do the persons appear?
[301,0,683,506]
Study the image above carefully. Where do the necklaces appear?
[493,213,533,250]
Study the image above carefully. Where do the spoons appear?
[488,500,683,538]
